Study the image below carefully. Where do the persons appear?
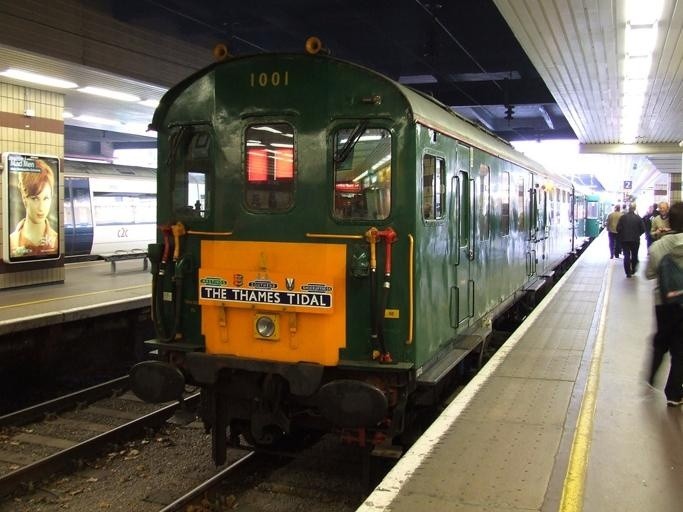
[605,202,683,407]
[10,160,59,257]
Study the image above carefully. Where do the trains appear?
[63,156,204,255]
[125,33,607,474]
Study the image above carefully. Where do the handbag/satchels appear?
[658,254,683,304]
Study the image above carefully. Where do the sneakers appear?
[667,398,683,406]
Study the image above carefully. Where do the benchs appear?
[105,254,149,273]
